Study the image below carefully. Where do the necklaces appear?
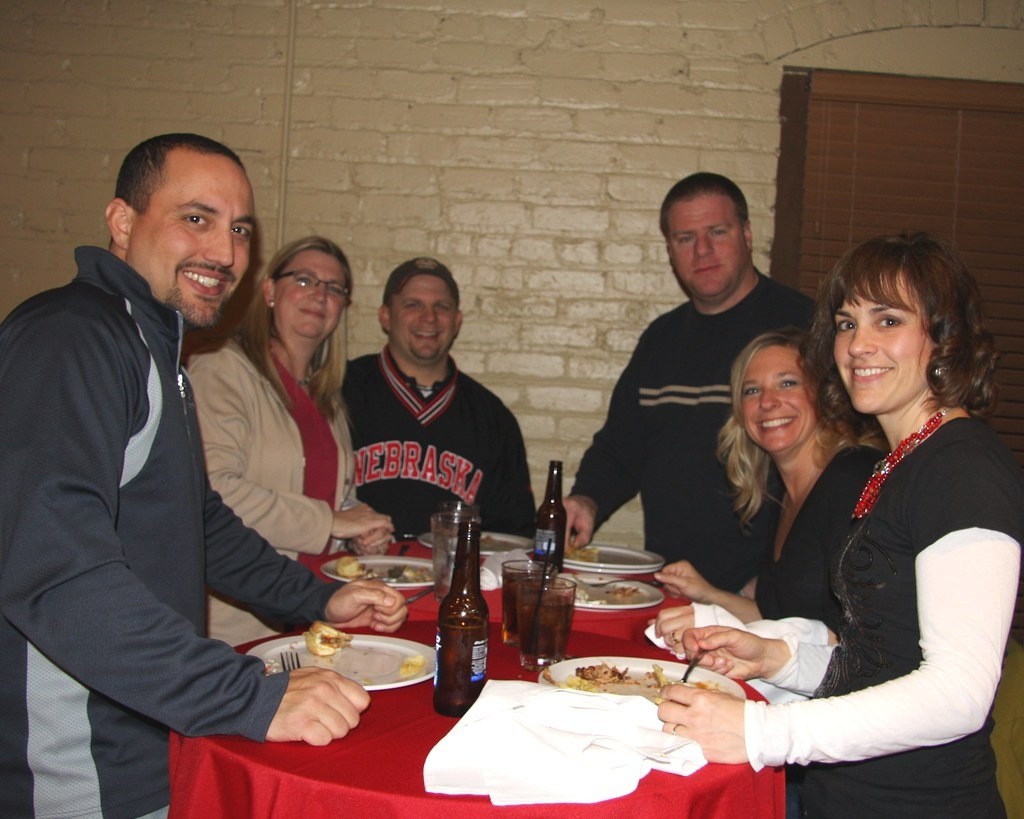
[300,367,313,385]
[418,385,432,391]
[852,407,949,518]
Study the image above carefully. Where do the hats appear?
[383,257,459,305]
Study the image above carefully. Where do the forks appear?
[279,652,301,671]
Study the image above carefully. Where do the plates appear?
[555,572,665,612]
[245,633,437,690]
[417,530,535,556]
[561,542,666,575]
[538,656,748,706]
[320,554,436,589]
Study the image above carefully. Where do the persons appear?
[562,172,818,593]
[657,236,1024,819]
[653,326,882,654]
[186,234,395,646]
[1,132,408,819]
[340,257,535,540]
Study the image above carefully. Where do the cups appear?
[430,500,480,600]
[514,576,577,673]
[501,560,553,647]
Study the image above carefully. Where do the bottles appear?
[432,515,490,717]
[533,459,568,569]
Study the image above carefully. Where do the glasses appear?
[275,269,348,296]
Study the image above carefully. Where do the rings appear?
[672,724,681,734]
[672,633,677,643]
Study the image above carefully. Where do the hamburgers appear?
[337,556,368,577]
[308,620,344,656]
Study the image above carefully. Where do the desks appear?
[176,534,787,819]
